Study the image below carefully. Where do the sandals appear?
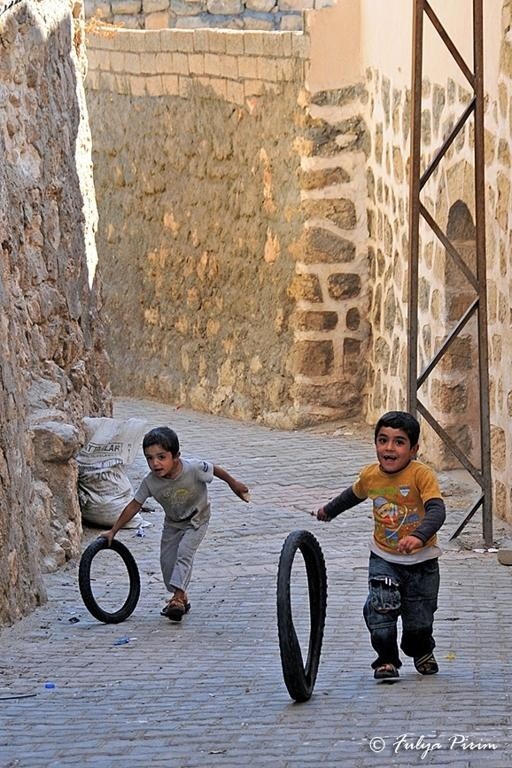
[374,663,399,680]
[160,596,191,616]
[166,597,185,621]
[414,651,439,675]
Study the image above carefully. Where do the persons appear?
[98,426,250,621]
[316,411,446,678]
